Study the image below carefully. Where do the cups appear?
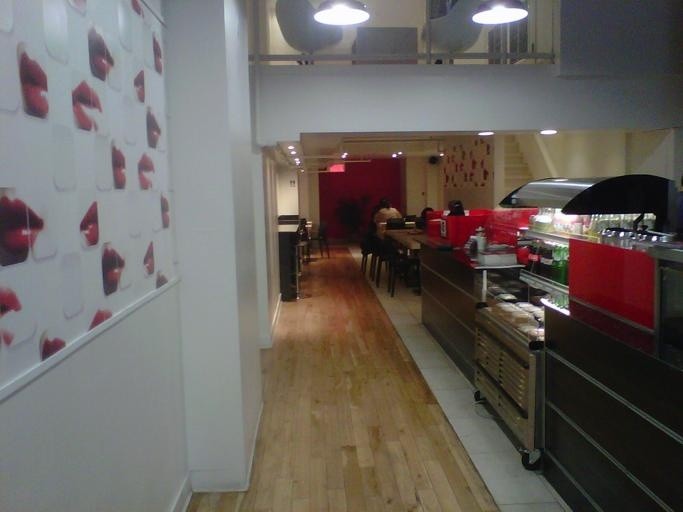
[475,236,486,253]
[600,229,669,253]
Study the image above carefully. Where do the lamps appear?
[314,0,369,28]
[474,0,527,25]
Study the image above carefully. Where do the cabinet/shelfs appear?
[500,175,669,300]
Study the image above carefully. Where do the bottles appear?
[534,207,654,238]
[474,225,485,237]
[524,240,567,282]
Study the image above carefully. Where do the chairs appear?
[277,215,422,296]
[423,0,483,65]
[275,0,343,65]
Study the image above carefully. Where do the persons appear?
[447,199,465,216]
[421,207,433,215]
[373,197,401,242]
